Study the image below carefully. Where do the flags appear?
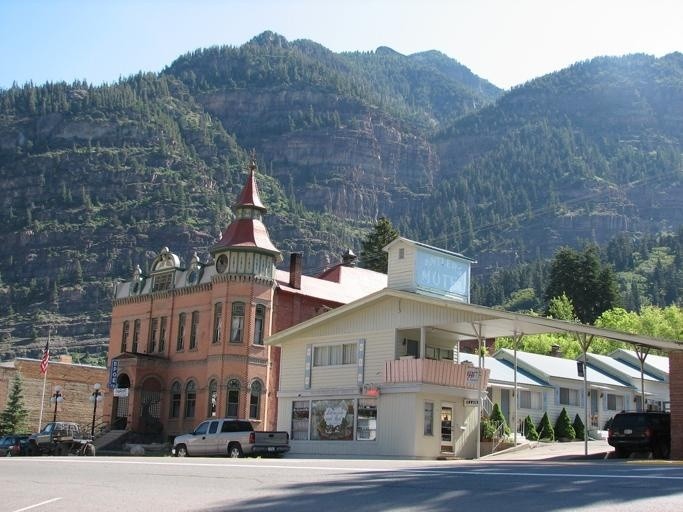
[39,341,48,376]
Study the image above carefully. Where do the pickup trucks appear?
[171,418,290,458]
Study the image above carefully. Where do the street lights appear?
[50,384,63,421]
[88,382,104,441]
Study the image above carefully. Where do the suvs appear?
[28,421,82,453]
[607,408,670,459]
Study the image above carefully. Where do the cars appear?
[0,434,43,457]
